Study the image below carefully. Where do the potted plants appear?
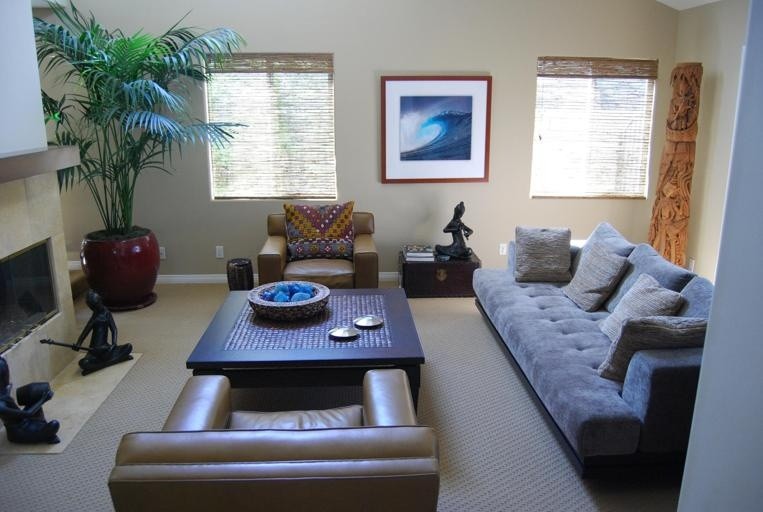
[32,0,248,314]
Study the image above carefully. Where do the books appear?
[402,244,435,262]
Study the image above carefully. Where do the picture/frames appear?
[380,76,492,183]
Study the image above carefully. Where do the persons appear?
[72,292,133,375]
[0,357,60,443]
[435,201,473,259]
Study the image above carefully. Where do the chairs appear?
[257,212,379,288]
[107,369,440,512]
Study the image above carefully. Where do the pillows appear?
[283,200,355,262]
[598,317,708,382]
[515,226,572,283]
[599,272,683,343]
[604,243,697,313]
[571,221,636,277]
[561,242,630,311]
[676,277,714,317]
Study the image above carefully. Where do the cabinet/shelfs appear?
[400,248,481,299]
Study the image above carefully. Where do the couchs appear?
[473,240,714,495]
